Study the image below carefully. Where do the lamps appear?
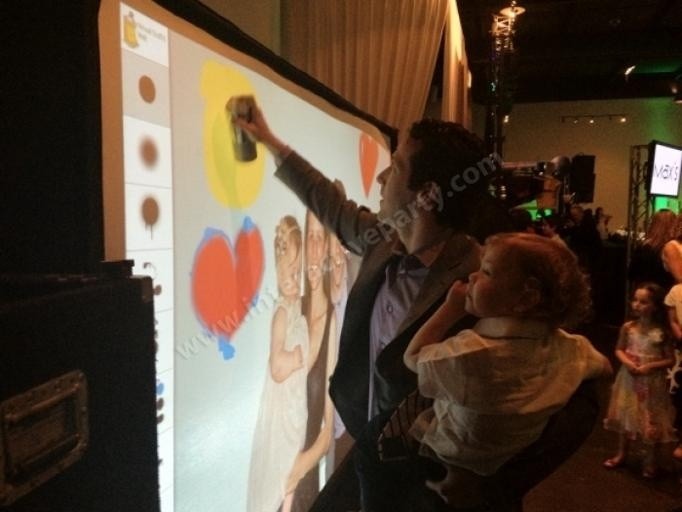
[537,154,571,179]
[500,0,527,17]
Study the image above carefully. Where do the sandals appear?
[643,463,655,478]
[603,451,626,467]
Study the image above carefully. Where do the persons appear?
[540,214,568,246]
[595,207,612,240]
[663,282,682,460]
[629,208,682,288]
[603,283,677,478]
[565,204,593,246]
[231,97,600,512]
[395,232,614,512]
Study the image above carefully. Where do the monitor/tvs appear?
[646,140,682,198]
